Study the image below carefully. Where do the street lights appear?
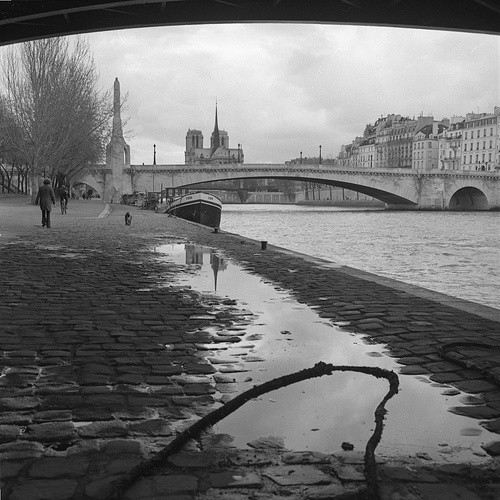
[153,143,156,165]
[319,144,322,165]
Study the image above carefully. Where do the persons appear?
[71,186,93,200]
[58,185,68,209]
[125,212,131,224]
[35,178,56,228]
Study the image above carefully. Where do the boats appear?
[166,190,223,227]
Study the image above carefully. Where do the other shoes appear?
[47,225,50,228]
[42,223,45,227]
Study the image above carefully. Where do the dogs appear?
[124,212,132,226]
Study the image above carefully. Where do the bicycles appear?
[60,196,68,215]
